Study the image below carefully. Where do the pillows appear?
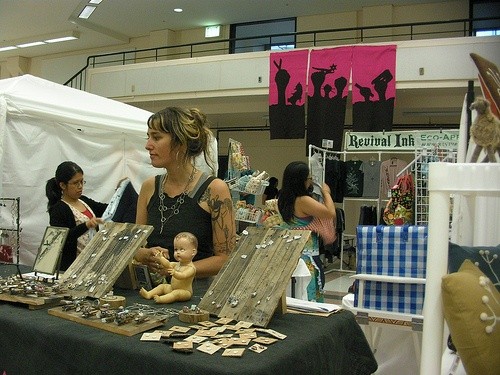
[448,241,500,293]
[441,259,500,375]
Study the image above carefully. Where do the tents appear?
[0,73,218,266]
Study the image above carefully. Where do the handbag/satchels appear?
[292,216,337,245]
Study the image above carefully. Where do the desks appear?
[0,264,379,375]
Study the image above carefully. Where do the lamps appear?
[0,30,80,51]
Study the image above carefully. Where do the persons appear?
[262,176,279,205]
[46,162,110,272]
[278,161,336,303]
[134,106,236,298]
[139,232,198,304]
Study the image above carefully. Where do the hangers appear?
[367,153,377,163]
[351,155,359,161]
[390,155,397,160]
[312,146,339,163]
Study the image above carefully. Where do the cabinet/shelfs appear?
[231,180,269,224]
[421,162,500,375]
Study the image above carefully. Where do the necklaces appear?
[158,165,198,234]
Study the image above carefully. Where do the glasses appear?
[307,177,312,181]
[63,180,86,185]
